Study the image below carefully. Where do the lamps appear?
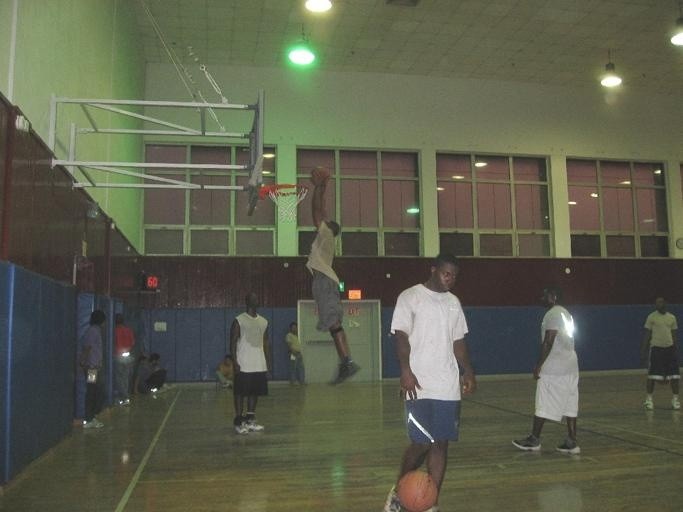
[670,17,683,46]
[601,48,622,88]
[288,23,315,65]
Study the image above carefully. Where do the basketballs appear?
[398,471,436,511]
[312,166,330,183]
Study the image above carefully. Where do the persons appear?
[306,170,362,387]
[382,253,475,512]
[78,310,104,428]
[512,282,581,454]
[641,298,681,411]
[285,322,307,386]
[215,354,235,388]
[230,293,272,434]
[113,312,170,405]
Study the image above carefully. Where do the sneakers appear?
[234,421,248,434]
[643,397,655,411]
[671,396,681,410]
[556,438,581,454]
[384,484,401,512]
[246,421,263,431]
[82,417,104,430]
[512,434,544,452]
[328,361,360,387]
